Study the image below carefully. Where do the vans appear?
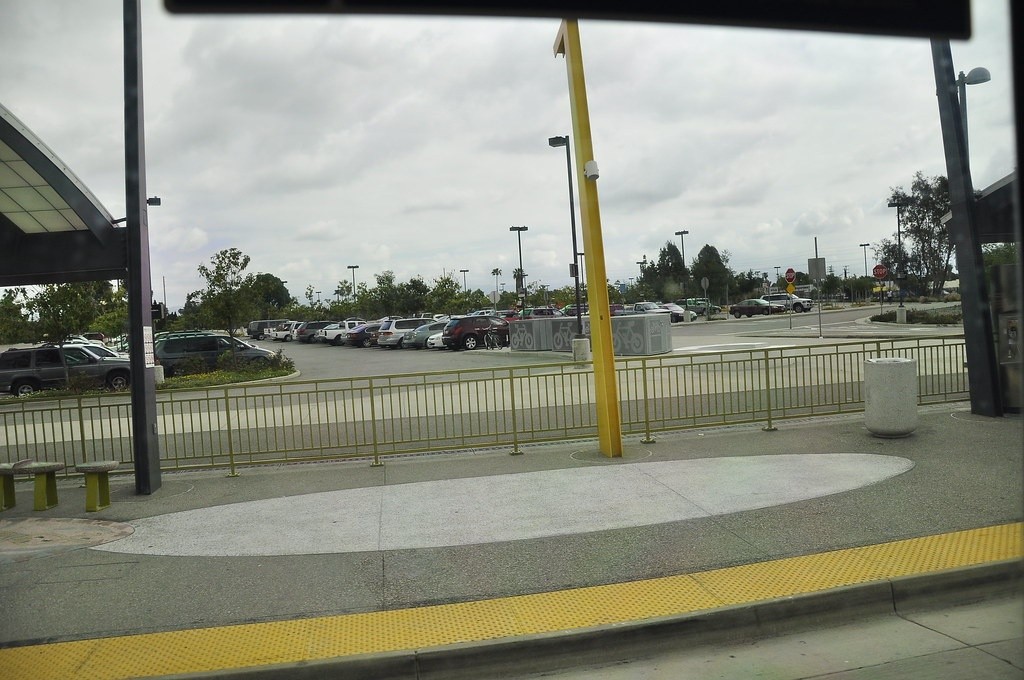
[247,319,291,340]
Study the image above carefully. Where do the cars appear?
[57,329,172,360]
[728,299,787,319]
[262,298,723,351]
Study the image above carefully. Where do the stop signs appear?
[872,265,887,279]
[784,268,797,284]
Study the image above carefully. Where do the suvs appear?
[760,293,814,314]
[0,343,131,397]
[154,331,280,379]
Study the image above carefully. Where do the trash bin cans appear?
[863,358,920,439]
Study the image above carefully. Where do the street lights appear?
[546,284,550,295]
[774,267,781,282]
[509,225,529,322]
[460,269,469,313]
[347,265,360,315]
[887,202,909,308]
[316,291,321,304]
[500,283,506,291]
[492,268,502,292]
[956,67,992,158]
[629,278,634,287]
[547,135,586,340]
[860,243,870,277]
[674,230,689,310]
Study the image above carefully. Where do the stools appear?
[76,461,119,512]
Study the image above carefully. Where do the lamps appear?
[583,161,600,180]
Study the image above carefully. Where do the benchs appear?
[0,459,65,511]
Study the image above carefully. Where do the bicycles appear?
[483,323,504,350]
